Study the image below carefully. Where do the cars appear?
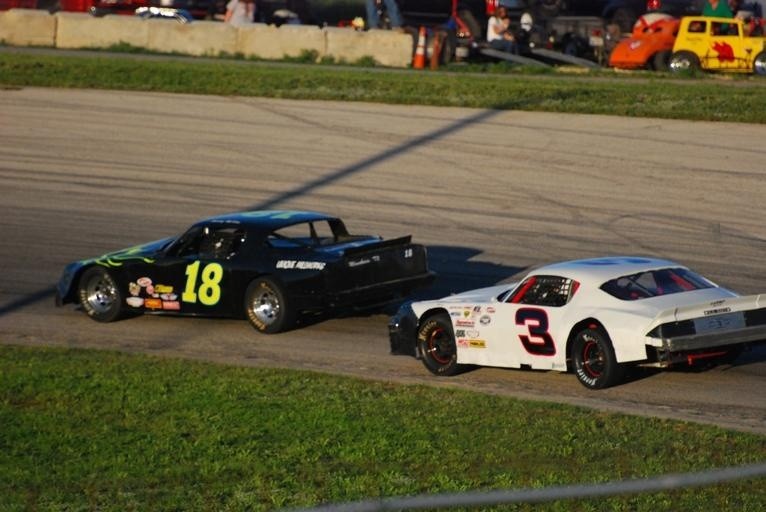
[548,10,765,79]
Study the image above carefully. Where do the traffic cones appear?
[429,29,441,71]
[411,24,428,71]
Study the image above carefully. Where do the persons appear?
[702,0,735,36]
[486,5,521,58]
[365,0,405,32]
[224,0,256,24]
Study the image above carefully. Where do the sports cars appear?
[51,209,435,337]
[386,254,765,388]
